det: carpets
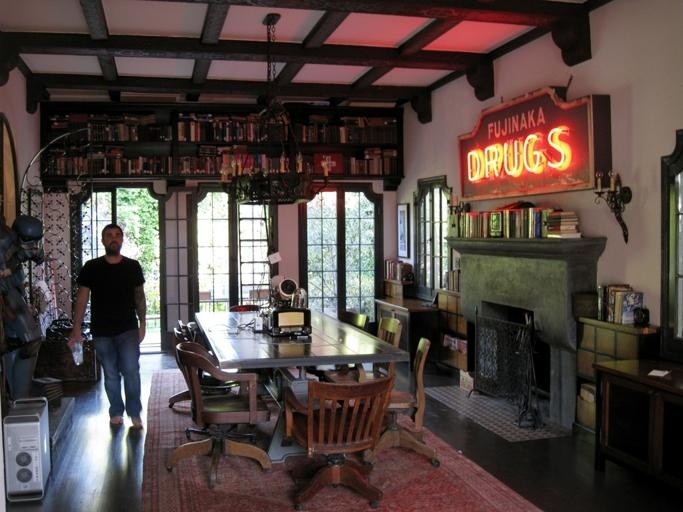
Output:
[140,368,544,512]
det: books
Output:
[443,270,459,293]
[48,111,399,180]
[384,258,412,282]
[595,284,645,326]
[448,207,581,239]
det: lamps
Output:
[228,14,328,205]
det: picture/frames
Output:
[396,203,410,259]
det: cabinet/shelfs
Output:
[593,360,683,488]
[573,315,658,446]
[375,298,439,372]
[436,287,476,376]
[382,280,416,298]
[41,101,404,180]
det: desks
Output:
[47,328,101,391]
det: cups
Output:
[70,341,83,366]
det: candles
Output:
[610,175,616,191]
[450,195,458,206]
[597,177,602,191]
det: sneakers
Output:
[131,416,143,430]
[110,416,123,425]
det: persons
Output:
[66,223,147,433]
